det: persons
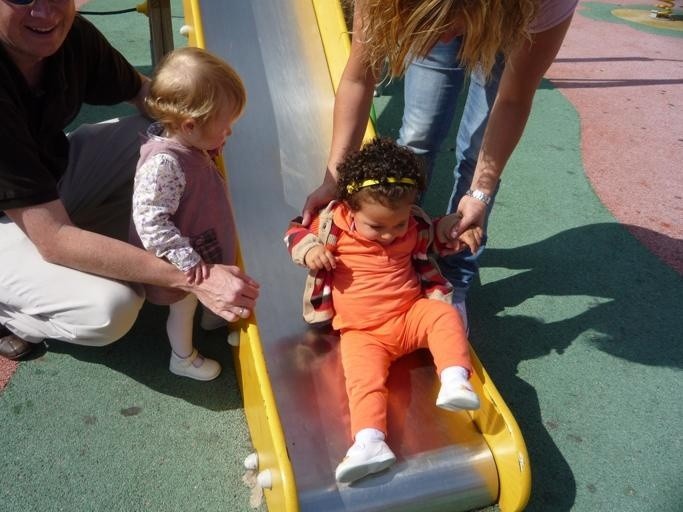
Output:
[279,135,487,486]
[114,45,248,384]
[298,1,580,341]
[0,0,262,378]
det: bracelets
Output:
[465,187,491,206]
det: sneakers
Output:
[452,298,471,340]
[333,437,397,486]
[435,380,483,413]
[0,333,36,359]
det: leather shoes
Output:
[166,347,223,382]
[198,316,227,333]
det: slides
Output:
[179,0,532,510]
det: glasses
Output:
[3,0,35,6]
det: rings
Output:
[237,307,244,317]
